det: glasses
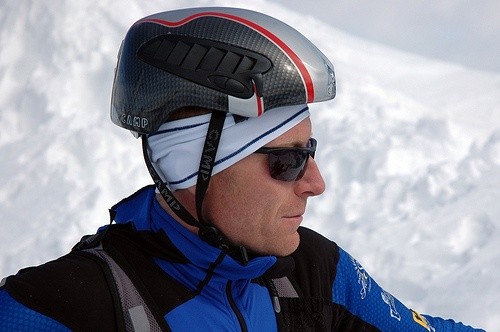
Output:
[247,139,318,183]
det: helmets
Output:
[110,6,338,132]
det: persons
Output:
[0,5,484,332]
